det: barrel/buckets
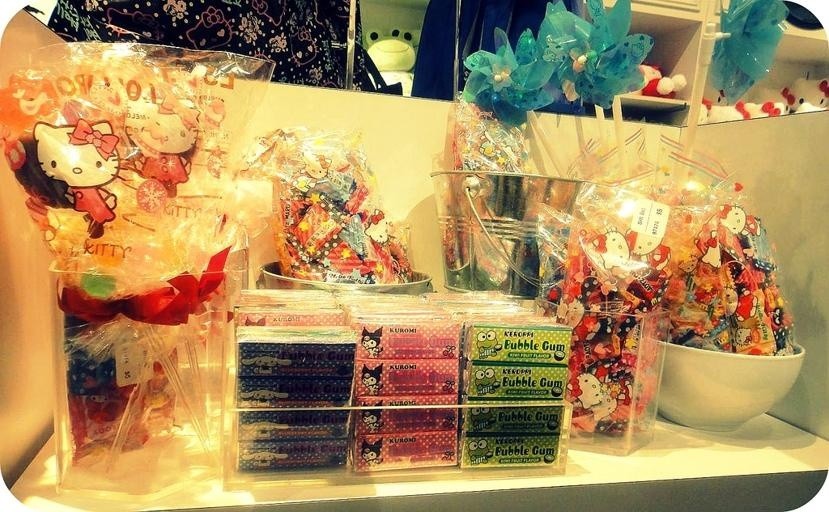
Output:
[430,170,582,303]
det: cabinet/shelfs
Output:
[579,0,828,125]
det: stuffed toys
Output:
[780,76,829,114]
[698,98,791,125]
[639,63,688,99]
[364,26,422,97]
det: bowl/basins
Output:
[655,339,805,431]
